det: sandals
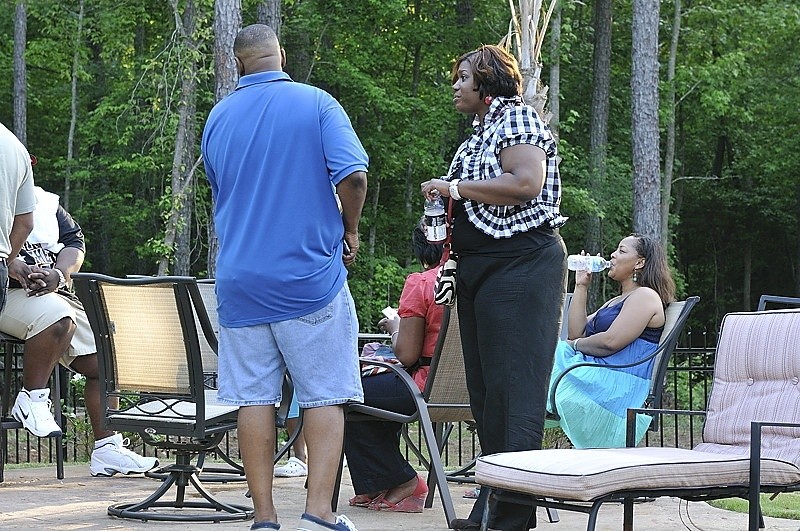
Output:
[273,457,307,477]
[463,490,480,499]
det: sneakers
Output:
[296,513,359,531]
[250,523,281,531]
[9,386,62,437]
[90,434,160,476]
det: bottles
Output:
[567,255,611,272]
[424,190,447,244]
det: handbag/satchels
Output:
[433,251,459,306]
[360,342,408,377]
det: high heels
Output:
[367,474,429,513]
[348,490,389,506]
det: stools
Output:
[0,334,65,481]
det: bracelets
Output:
[573,338,581,351]
[391,331,400,342]
[449,179,463,200]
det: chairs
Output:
[71,265,800,531]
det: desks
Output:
[303,335,390,515]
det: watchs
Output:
[53,268,66,288]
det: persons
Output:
[0,153,160,477]
[544,233,676,449]
[201,23,369,531]
[0,122,36,317]
[344,219,444,512]
[421,46,568,531]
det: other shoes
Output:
[451,518,481,531]
